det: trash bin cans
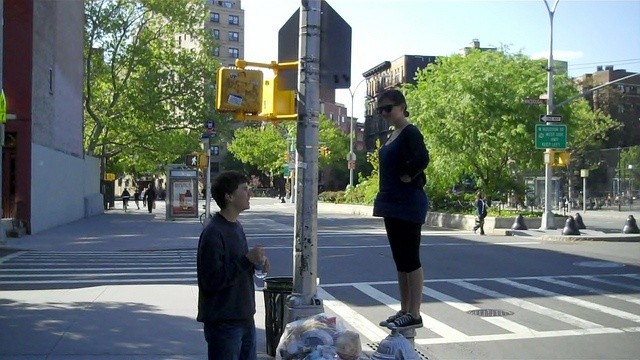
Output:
[263,276,294,357]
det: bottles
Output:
[252,260,267,292]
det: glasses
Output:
[375,104,395,114]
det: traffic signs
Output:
[538,114,563,124]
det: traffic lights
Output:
[184,154,198,167]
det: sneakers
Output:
[472,228,476,234]
[380,310,407,327]
[481,234,486,236]
[387,313,423,331]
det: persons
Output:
[195,171,267,360]
[371,89,430,330]
[121,182,156,216]
[608,191,625,206]
[473,191,487,235]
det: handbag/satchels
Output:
[475,218,482,225]
